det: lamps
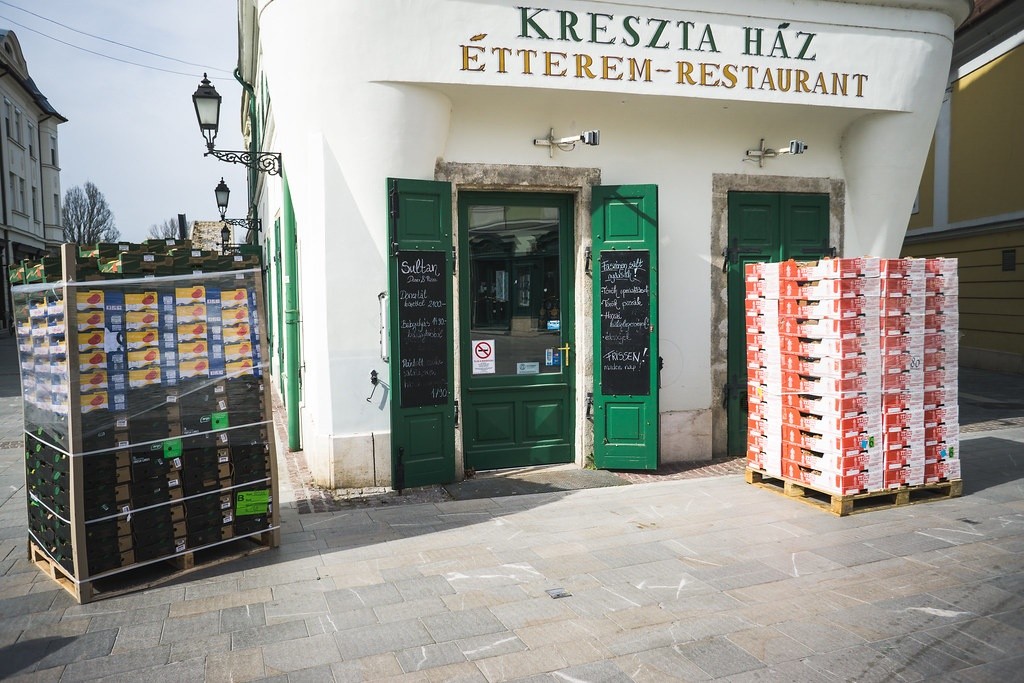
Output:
[535,129,601,149]
[193,72,282,175]
[746,138,809,166]
[220,223,240,255]
[214,177,262,233]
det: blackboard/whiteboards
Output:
[398,251,449,407]
[600,250,650,395]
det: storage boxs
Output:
[742,254,960,495]
[7,237,274,576]
